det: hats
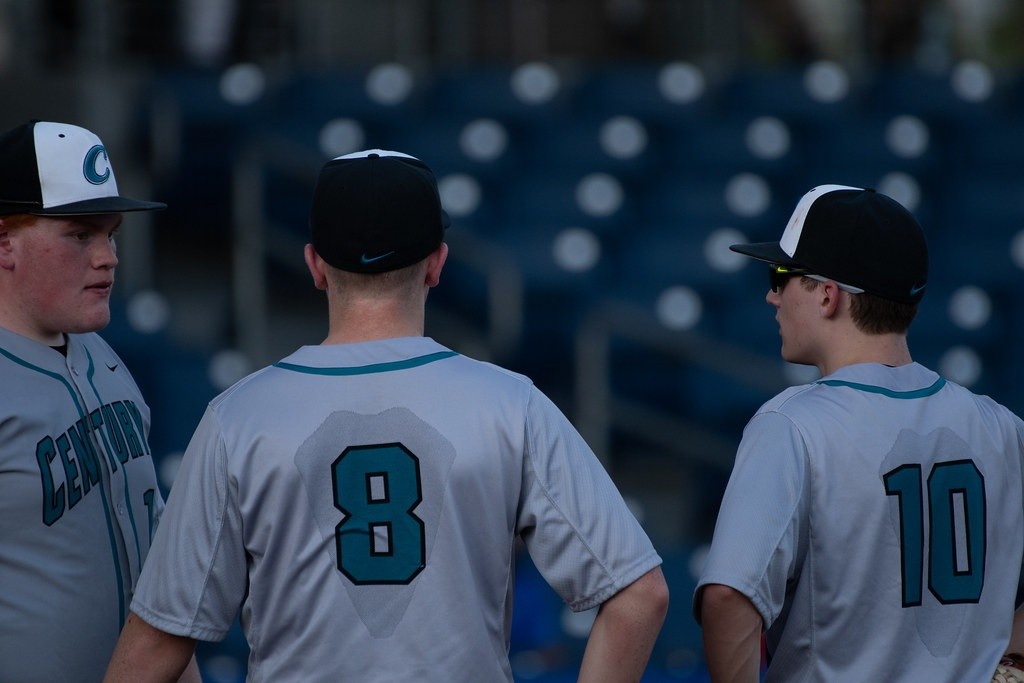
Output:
[730,182,928,305]
[0,119,167,215]
[309,149,448,273]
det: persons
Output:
[690,184,1024,683]
[0,122,202,683]
[102,149,670,683]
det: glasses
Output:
[767,262,817,294]
